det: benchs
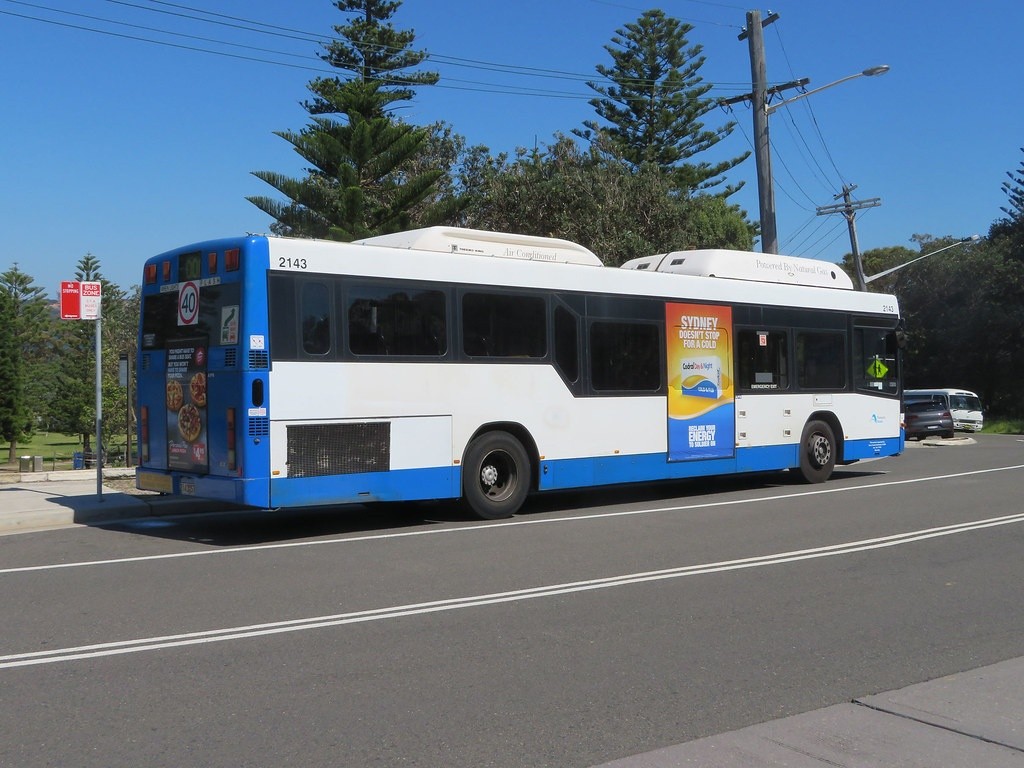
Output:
[73,452,107,469]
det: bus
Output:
[134,226,915,514]
[903,390,984,433]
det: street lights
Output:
[752,65,890,253]
[855,235,982,293]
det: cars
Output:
[902,399,954,441]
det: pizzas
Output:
[167,380,183,410]
[178,404,201,441]
[190,373,206,406]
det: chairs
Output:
[364,333,491,355]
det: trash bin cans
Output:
[73,453,85,470]
[19,456,30,472]
[32,455,43,472]
